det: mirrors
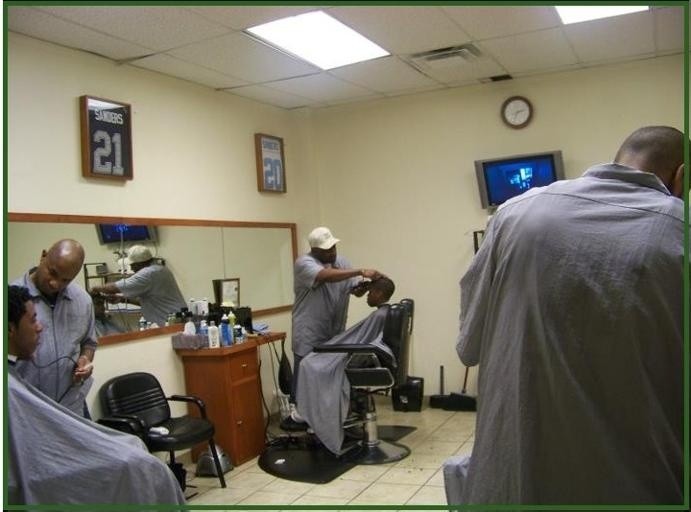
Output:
[8,211,298,348]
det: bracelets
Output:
[361,266,365,274]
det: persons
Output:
[292,227,382,439]
[8,286,43,367]
[444,125,691,512]
[367,277,395,306]
[9,238,100,420]
[92,293,105,320]
[92,244,187,328]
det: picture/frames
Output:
[252,131,289,196]
[78,94,134,180]
[217,278,241,311]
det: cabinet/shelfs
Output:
[80,256,167,315]
[173,330,289,468]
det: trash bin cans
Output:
[391,377,424,411]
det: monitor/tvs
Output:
[474,150,562,208]
[95,223,156,245]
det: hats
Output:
[307,226,339,251]
[117,245,153,264]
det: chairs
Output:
[99,371,229,491]
[282,296,416,464]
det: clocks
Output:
[500,94,535,131]
